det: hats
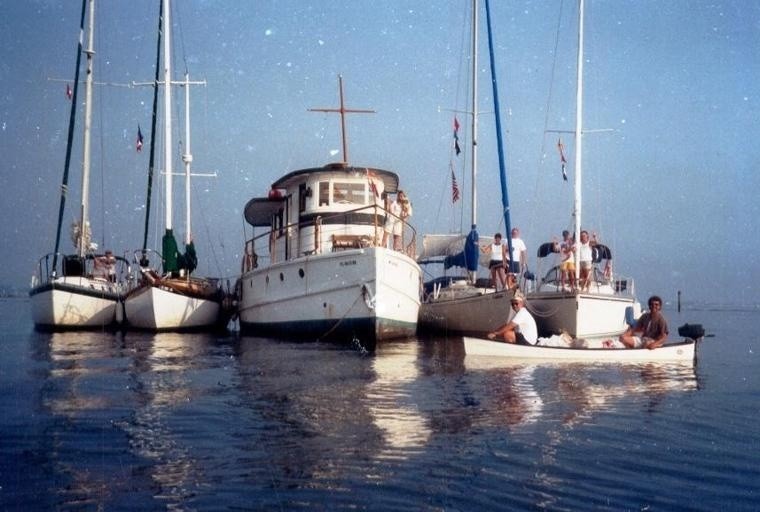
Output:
[511,294,524,301]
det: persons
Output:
[619,296,669,349]
[553,230,576,292]
[95,249,116,283]
[480,233,508,293]
[488,296,538,345]
[382,191,409,251]
[504,228,526,288]
[478,361,677,437]
[572,231,598,292]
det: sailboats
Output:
[416,0,533,339]
[23,0,123,332]
[121,0,229,333]
[525,1,645,341]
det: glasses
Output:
[511,302,517,306]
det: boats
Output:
[230,73,422,355]
[460,334,696,364]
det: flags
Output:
[136,124,143,151]
[454,118,460,129]
[562,165,569,181]
[67,83,74,99]
[558,141,568,163]
[450,165,459,204]
[370,178,380,199]
[453,130,460,139]
[453,141,461,155]
